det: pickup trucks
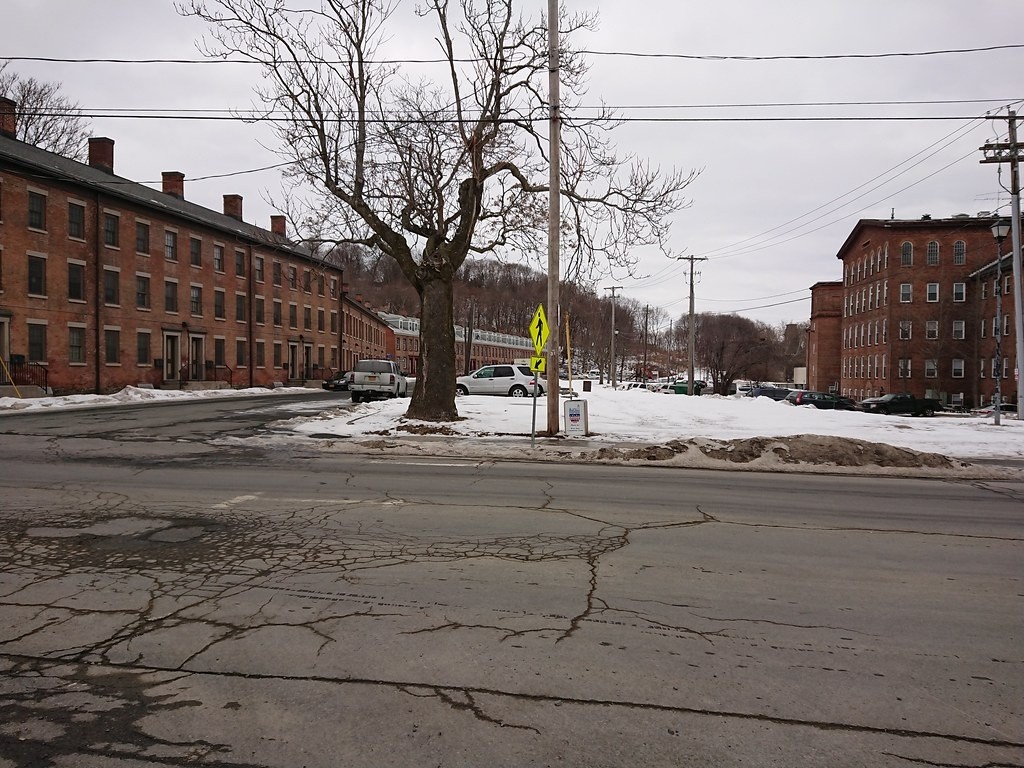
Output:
[857,393,944,417]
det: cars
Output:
[970,403,1017,417]
[455,364,546,398]
[628,383,675,394]
[747,388,857,411]
[322,358,408,402]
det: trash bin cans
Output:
[583,381,591,392]
[674,382,688,394]
[564,400,588,436]
